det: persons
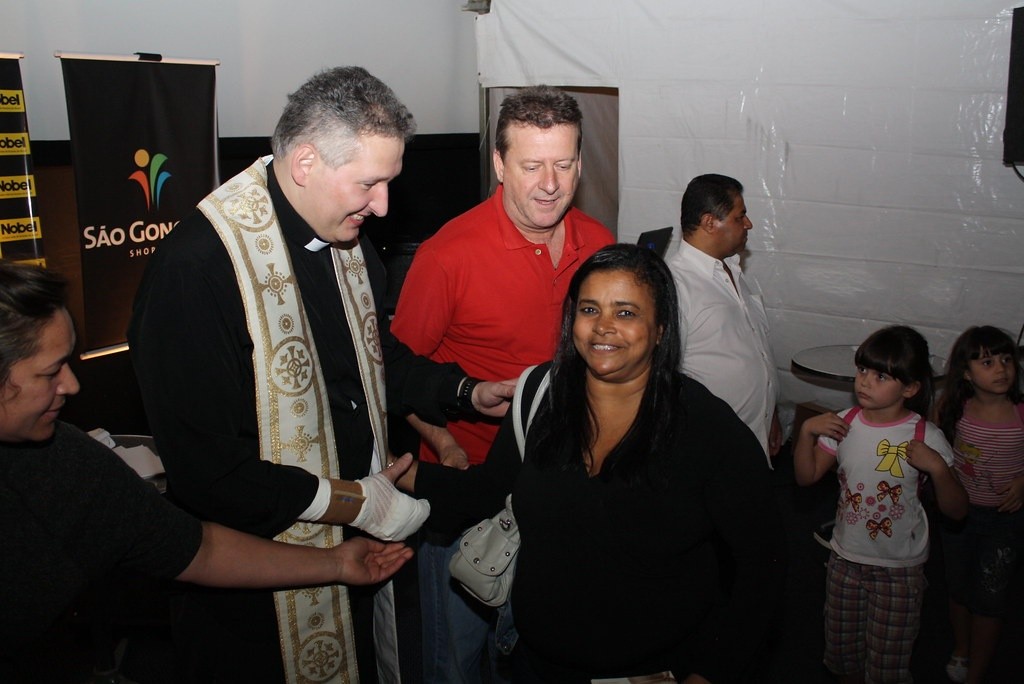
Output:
[932,326,1024,684]
[391,90,616,684]
[0,259,414,684]
[384,245,773,684]
[794,324,963,684]
[661,173,786,466]
[127,70,519,684]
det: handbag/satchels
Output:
[449,497,520,607]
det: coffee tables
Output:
[791,345,952,384]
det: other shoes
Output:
[947,655,970,682]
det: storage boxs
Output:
[790,398,845,446]
